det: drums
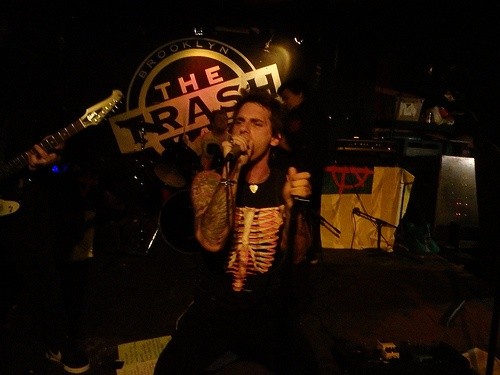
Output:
[159,190,204,255]
[152,139,200,188]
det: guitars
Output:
[0,90,125,180]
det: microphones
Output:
[226,145,242,162]
